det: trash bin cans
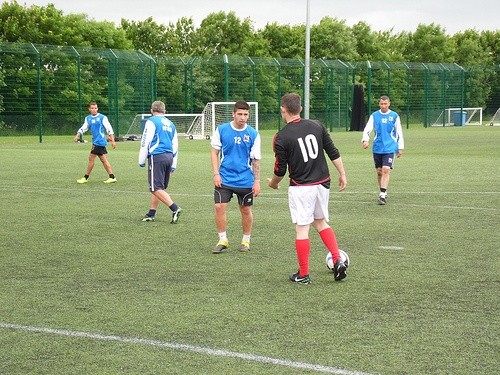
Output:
[454,111,467,126]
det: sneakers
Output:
[334,258,346,281]
[170,207,181,224]
[77,177,88,183]
[378,197,386,204]
[289,271,311,285]
[141,214,154,221]
[240,241,249,253]
[212,241,228,253]
[104,178,117,184]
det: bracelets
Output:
[213,174,219,176]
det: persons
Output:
[361,95,404,205]
[211,100,261,253]
[138,101,182,224]
[74,101,117,184]
[267,93,347,285]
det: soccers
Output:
[326,249,350,273]
